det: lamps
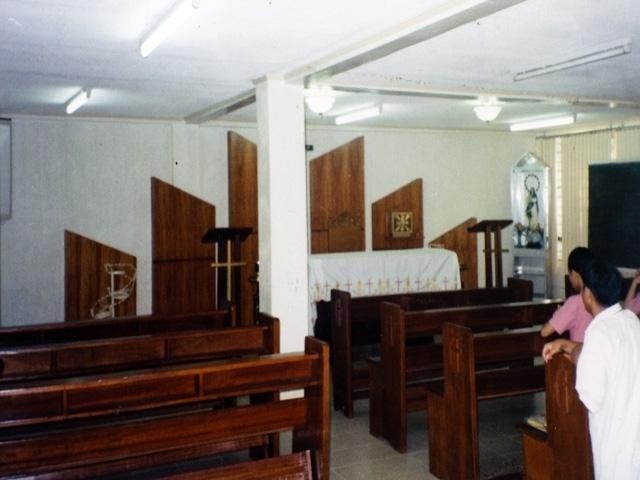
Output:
[509,111,575,132]
[139,0,196,58]
[334,101,382,125]
[65,84,92,115]
[473,106,502,124]
[513,37,631,82]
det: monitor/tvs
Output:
[586,160,640,280]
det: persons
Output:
[525,187,539,232]
[540,247,640,480]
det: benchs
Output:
[0,274,640,480]
